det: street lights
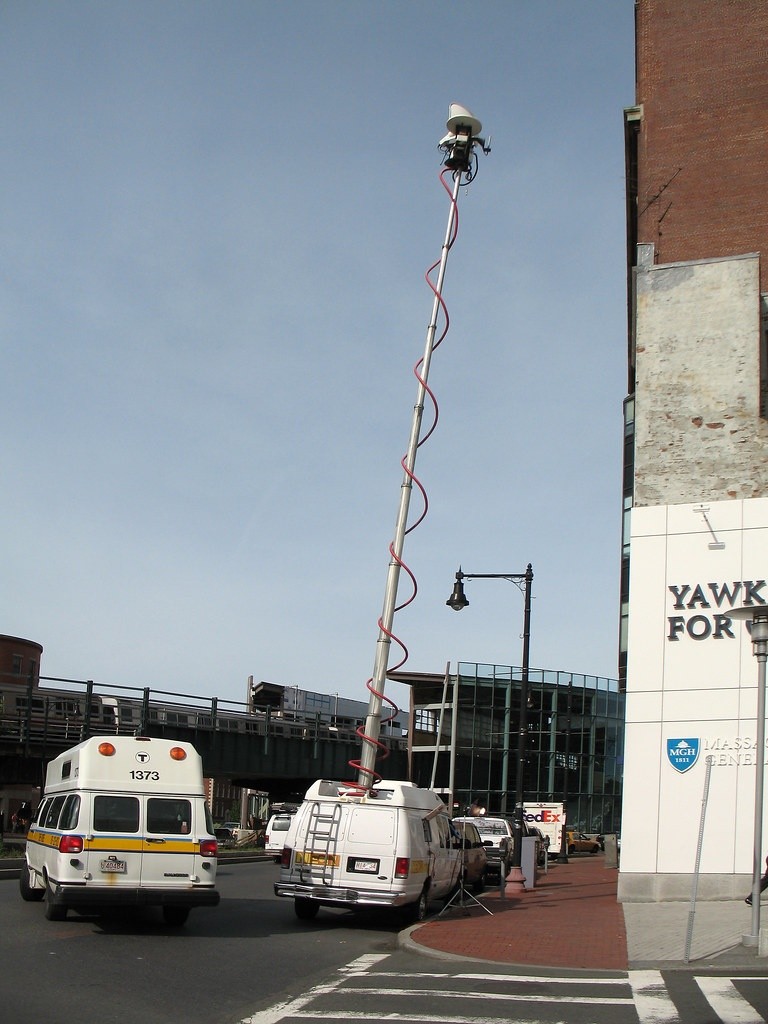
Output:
[447,571,535,823]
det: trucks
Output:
[515,801,567,859]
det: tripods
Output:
[435,807,494,917]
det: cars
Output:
[214,828,236,849]
[601,834,621,851]
[222,822,240,838]
[565,832,602,854]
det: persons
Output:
[11,812,18,833]
[745,857,768,907]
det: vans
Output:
[262,813,295,863]
[20,733,221,923]
[273,779,471,926]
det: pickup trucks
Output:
[452,816,516,873]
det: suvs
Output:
[449,822,494,893]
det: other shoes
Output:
[745,898,752,905]
